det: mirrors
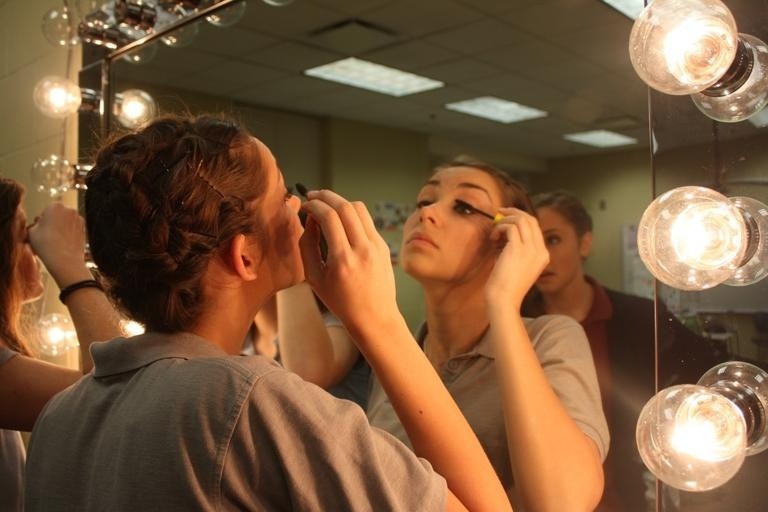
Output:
[75,0,768,511]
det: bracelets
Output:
[56,280,105,303]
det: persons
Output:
[365,151,612,512]
[522,188,715,511]
[21,109,513,511]
[237,277,372,414]
[0,178,130,512]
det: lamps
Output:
[262,1,296,7]
[689,33,768,124]
[635,186,750,292]
[635,384,755,492]
[720,196,768,286]
[205,1,248,28]
[628,0,743,97]
[695,361,768,457]
